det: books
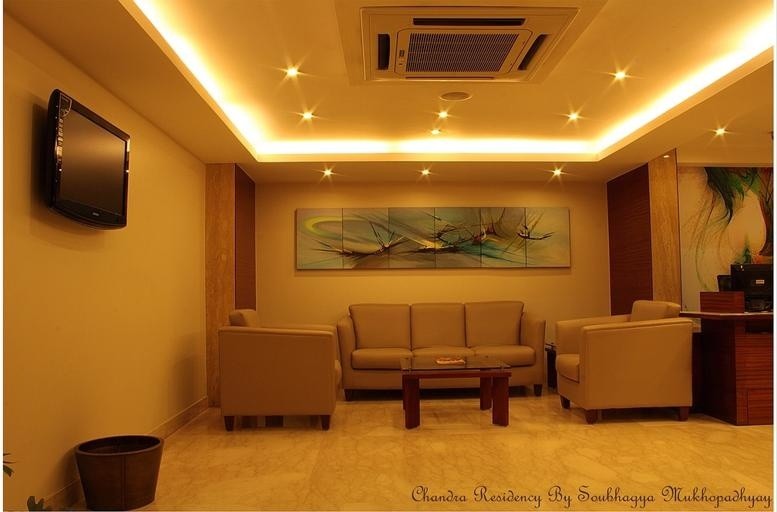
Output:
[434,359,465,364]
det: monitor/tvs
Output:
[46,89,131,231]
[731,263,773,312]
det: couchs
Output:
[337,300,546,401]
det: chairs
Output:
[554,299,693,425]
[217,308,341,429]
